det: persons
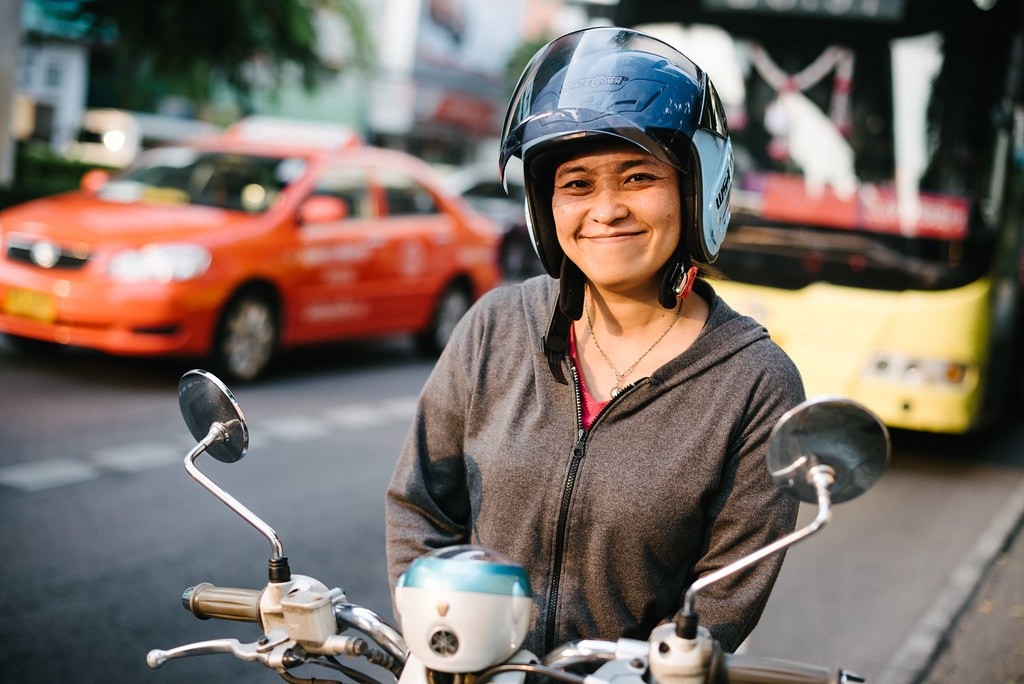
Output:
[379,25,808,684]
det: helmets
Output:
[521,52,735,276]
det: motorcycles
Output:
[144,369,889,684]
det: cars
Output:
[0,118,508,383]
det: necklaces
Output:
[583,278,683,403]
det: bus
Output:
[608,1,1023,435]
